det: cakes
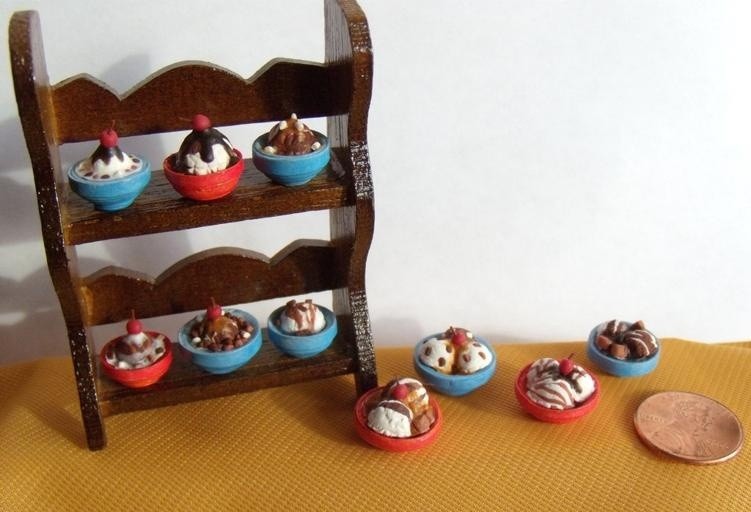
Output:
[106,310,166,369]
[171,115,237,174]
[365,375,434,438]
[525,357,596,408]
[274,299,326,336]
[264,113,320,156]
[596,318,658,359]
[191,297,252,350]
[75,119,142,181]
[420,327,492,374]
